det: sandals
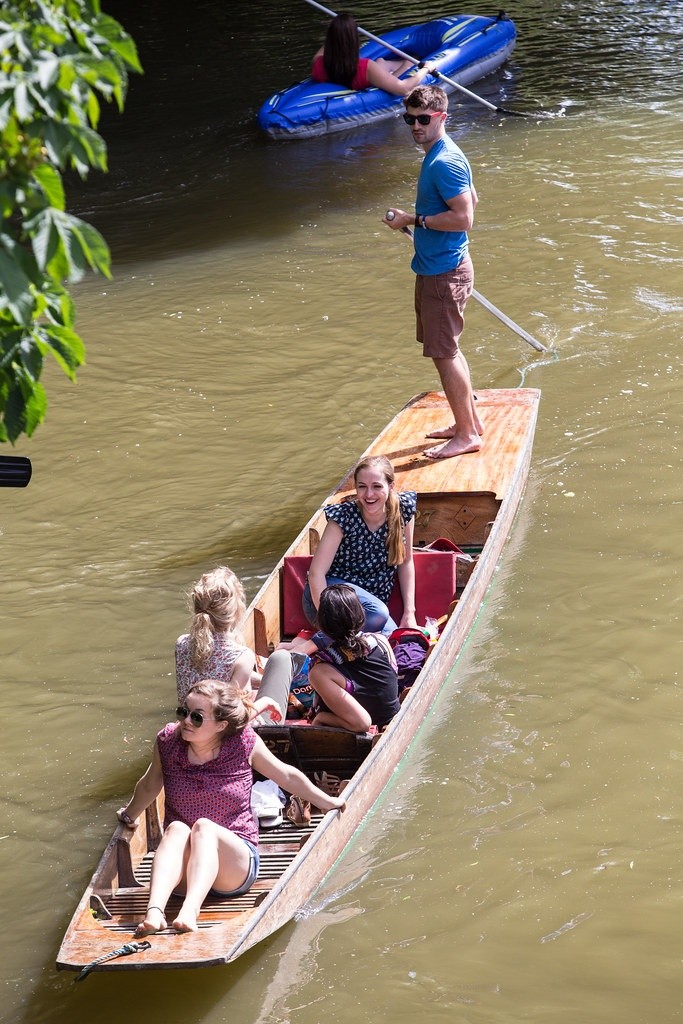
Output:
[285,795,311,828]
[314,771,341,814]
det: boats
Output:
[54,386,542,969]
[256,14,518,139]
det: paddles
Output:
[304,0,535,118]
[386,210,547,352]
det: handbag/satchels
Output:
[389,627,429,687]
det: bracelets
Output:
[422,215,428,230]
[414,213,423,228]
[423,67,430,73]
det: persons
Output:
[309,13,437,95]
[175,566,295,727]
[275,455,417,655]
[309,584,402,734]
[115,679,347,933]
[380,85,485,459]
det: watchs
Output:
[121,810,134,824]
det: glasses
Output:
[403,112,442,125]
[176,706,220,728]
